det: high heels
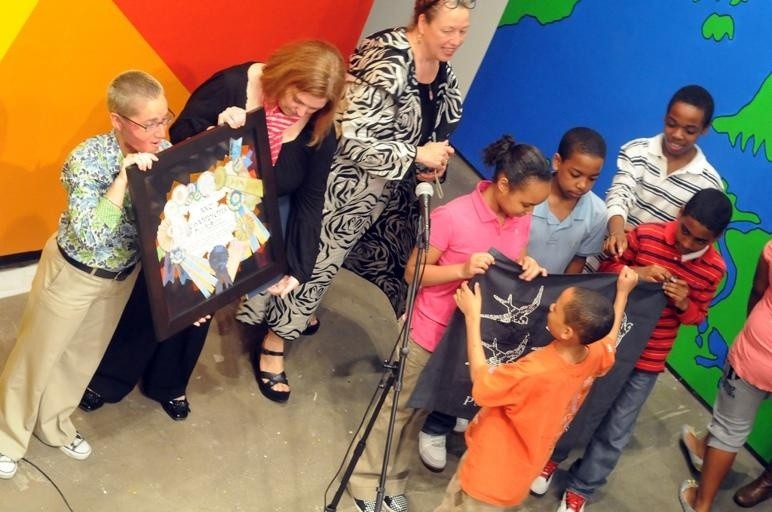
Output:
[252,338,291,402]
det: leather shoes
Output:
[79,388,104,412]
[162,394,190,420]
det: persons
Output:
[530,188,733,512]
[79,40,346,420]
[734,460,772,508]
[680,240,772,511]
[343,132,550,512]
[0,68,175,478]
[431,266,638,512]
[237,0,476,403]
[420,126,606,472]
[603,84,726,258]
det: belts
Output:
[57,241,142,282]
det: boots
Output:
[733,458,770,508]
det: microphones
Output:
[415,182,433,250]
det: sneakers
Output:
[418,429,447,470]
[0,453,19,480]
[555,489,587,512]
[453,417,470,434]
[58,430,92,460]
[353,495,387,512]
[679,478,700,512]
[383,494,411,512]
[531,459,557,496]
[681,422,703,472]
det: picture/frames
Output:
[126,105,290,343]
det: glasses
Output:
[116,107,176,132]
[420,0,477,13]
